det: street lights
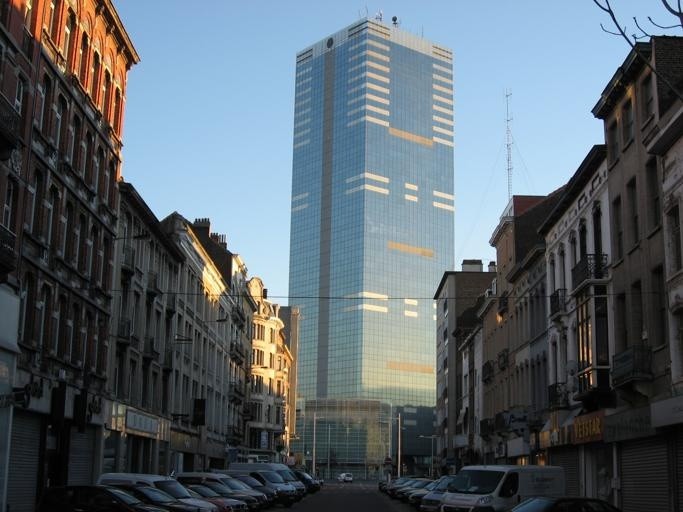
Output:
[416,433,437,479]
[377,421,390,463]
[312,411,325,478]
[387,412,401,479]
[326,425,336,482]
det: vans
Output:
[336,473,354,483]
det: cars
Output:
[378,463,621,512]
[40,459,325,512]
